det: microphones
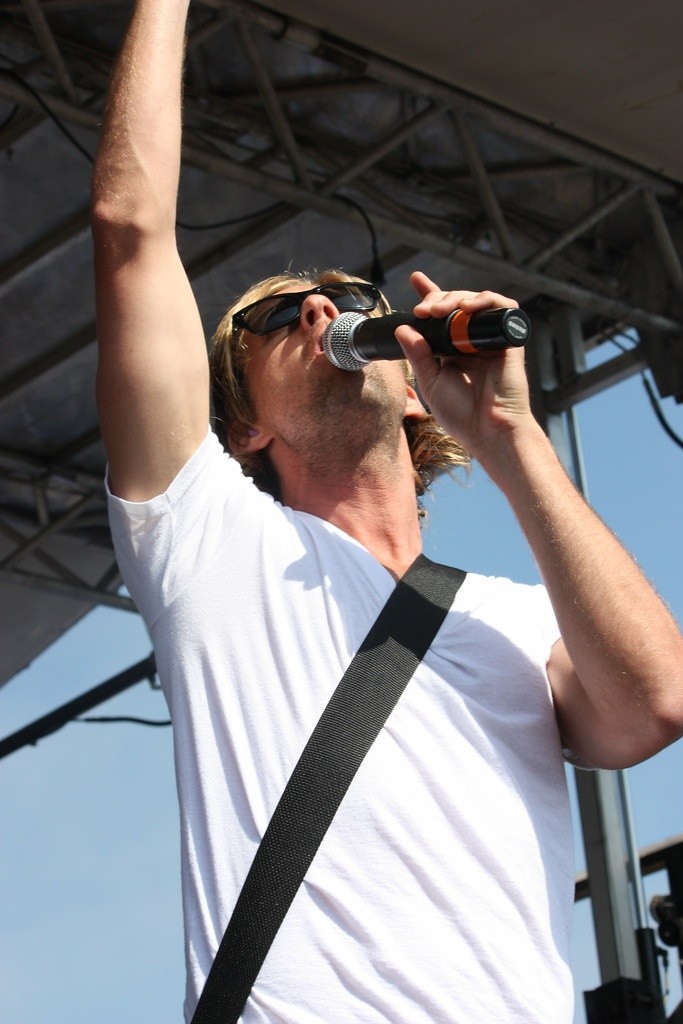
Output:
[322,311,529,371]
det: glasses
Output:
[230,281,387,374]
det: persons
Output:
[91,1,683,1023]
